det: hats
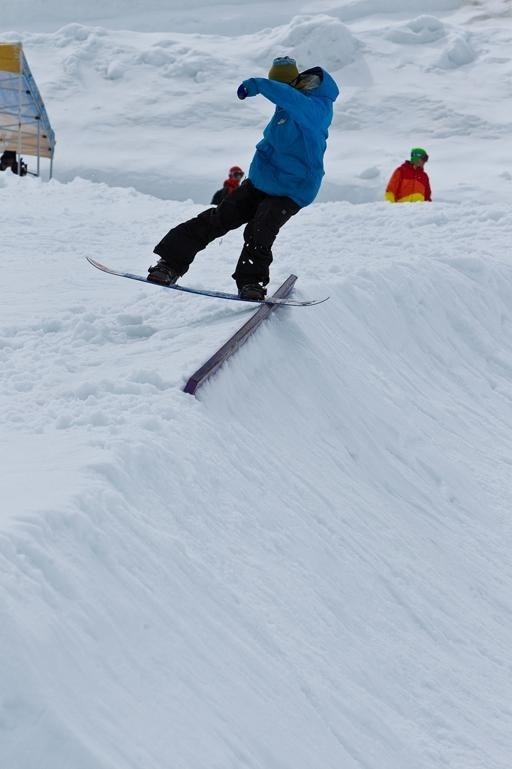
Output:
[228,167,243,179]
[268,56,299,85]
[411,148,427,164]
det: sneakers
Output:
[147,262,175,285]
[238,281,267,301]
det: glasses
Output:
[232,172,244,178]
[419,154,428,162]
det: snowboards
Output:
[86,256,329,306]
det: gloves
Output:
[237,84,247,101]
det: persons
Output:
[147,54,340,301]
[210,166,244,205]
[384,147,432,203]
